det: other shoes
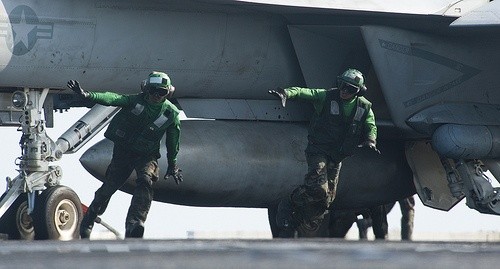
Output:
[126,225,144,237]
[81,215,94,238]
[276,198,295,228]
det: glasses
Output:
[340,82,356,93]
[150,86,167,95]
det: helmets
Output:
[336,69,367,97]
[141,71,172,97]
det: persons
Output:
[264,69,381,234]
[368,196,415,242]
[66,70,184,239]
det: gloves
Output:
[67,79,87,99]
[357,139,382,160]
[164,165,184,184]
[265,87,287,108]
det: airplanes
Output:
[0,0,500,243]
